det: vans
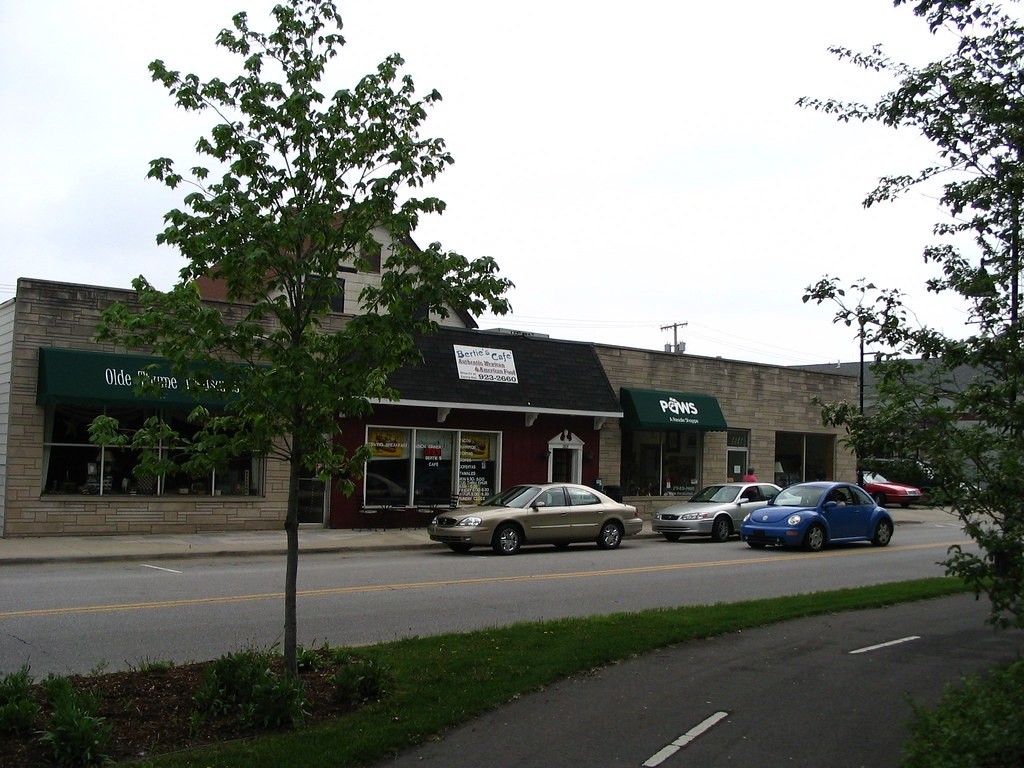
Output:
[858,459,939,504]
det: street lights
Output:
[858,314,868,412]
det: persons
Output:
[827,488,847,508]
[741,466,759,494]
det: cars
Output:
[298,473,408,521]
[858,470,922,507]
[651,482,802,542]
[428,482,643,556]
[740,480,894,553]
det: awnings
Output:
[33,348,280,420]
[619,387,727,435]
